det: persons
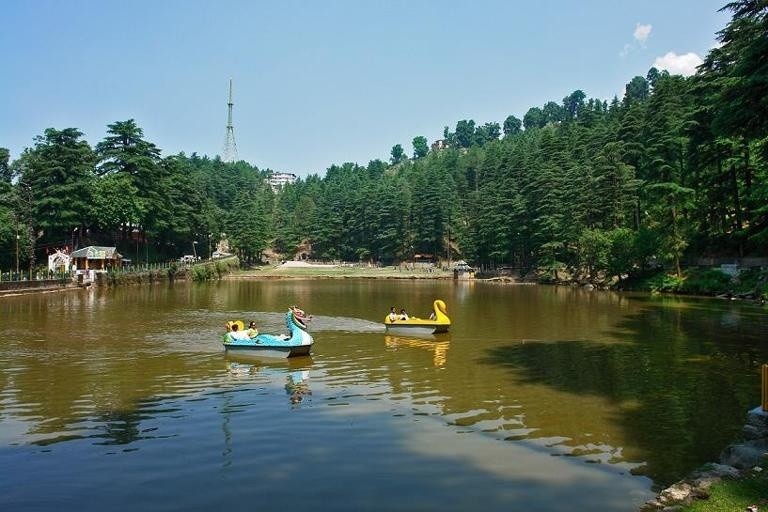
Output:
[247,321,258,339]
[389,307,397,321]
[429,308,437,321]
[399,309,409,320]
[229,324,250,340]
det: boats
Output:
[383,299,452,334]
[221,305,314,358]
[225,354,313,411]
[385,334,451,370]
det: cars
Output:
[212,252,221,259]
